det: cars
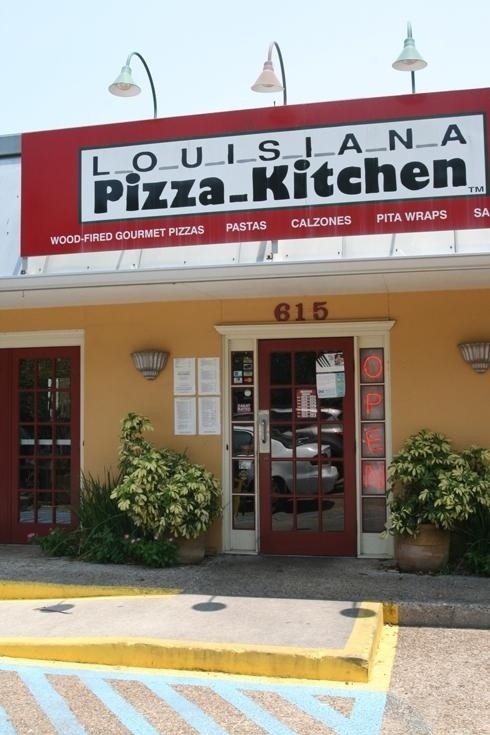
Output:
[232,405,344,485]
[233,425,339,514]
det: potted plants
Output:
[377,427,489,572]
[108,411,224,566]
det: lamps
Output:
[108,50,159,119]
[250,39,287,105]
[392,21,428,93]
